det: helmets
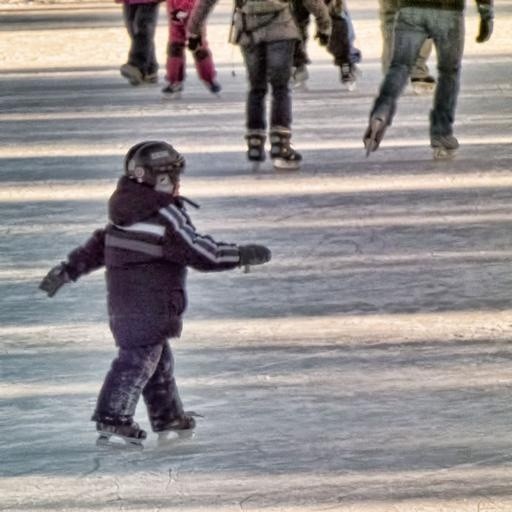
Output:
[124,141,185,195]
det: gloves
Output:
[39,261,71,297]
[239,244,271,273]
[476,3,495,43]
[313,29,332,46]
[188,33,203,50]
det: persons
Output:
[38,139,272,452]
[377,0,437,96]
[288,1,362,92]
[162,0,223,97]
[116,0,162,86]
[227,1,310,171]
[362,0,495,161]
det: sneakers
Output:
[288,63,309,84]
[363,102,392,152]
[432,133,459,150]
[270,132,302,162]
[152,414,196,433]
[143,72,158,83]
[96,421,148,439]
[411,77,436,87]
[247,135,266,160]
[162,82,185,93]
[339,60,359,82]
[207,81,222,92]
[120,64,142,86]
